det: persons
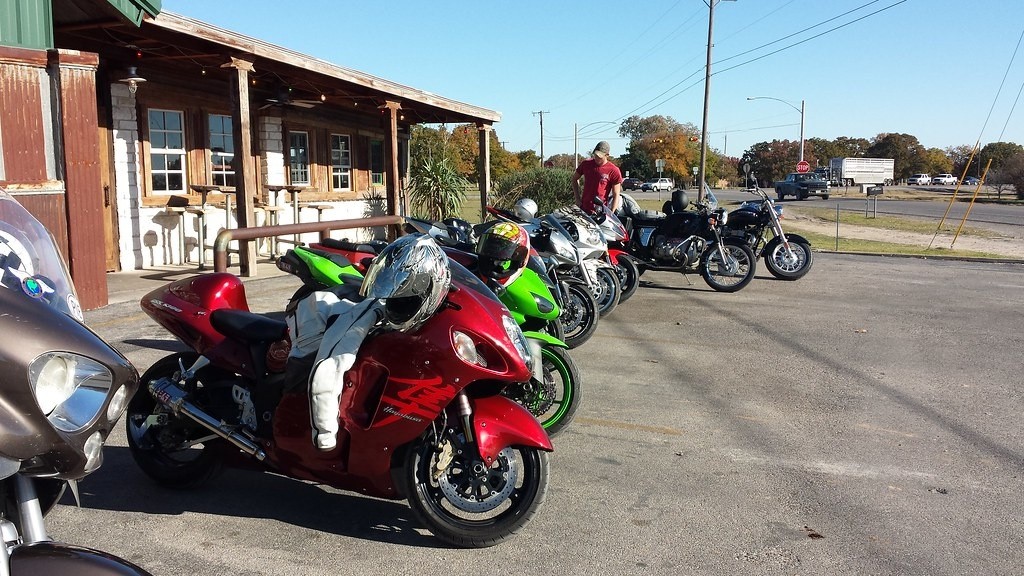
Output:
[571,140,622,216]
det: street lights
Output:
[574,120,617,180]
[748,95,812,169]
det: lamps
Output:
[118,66,148,94]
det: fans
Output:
[257,93,324,110]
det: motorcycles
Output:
[278,161,815,447]
[124,234,556,549]
[0,191,155,574]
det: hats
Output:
[592,141,610,159]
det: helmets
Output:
[672,190,689,210]
[514,198,539,223]
[476,221,530,288]
[358,232,451,335]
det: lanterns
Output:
[689,136,698,142]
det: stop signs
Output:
[795,161,810,173]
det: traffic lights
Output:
[651,138,663,143]
[689,136,697,141]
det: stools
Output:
[166,202,334,264]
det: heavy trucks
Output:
[818,157,895,187]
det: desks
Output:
[190,184,236,267]
[264,185,306,261]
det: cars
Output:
[621,178,644,190]
[961,176,984,186]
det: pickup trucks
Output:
[908,174,936,185]
[932,174,958,185]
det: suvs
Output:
[773,172,830,201]
[642,177,675,192]
